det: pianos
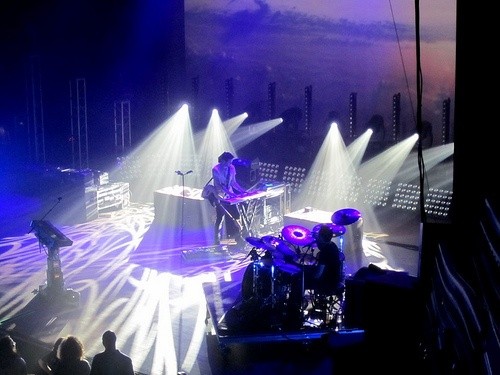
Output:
[219,190,267,251]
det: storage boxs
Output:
[42,170,129,223]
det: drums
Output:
[279,245,297,255]
[242,263,273,300]
[265,250,284,259]
[274,264,302,311]
[292,253,318,266]
[260,259,285,266]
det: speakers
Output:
[231,160,256,193]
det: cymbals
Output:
[331,209,360,225]
[313,223,346,238]
[282,225,314,246]
[262,235,282,249]
[245,237,270,249]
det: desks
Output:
[154,181,334,240]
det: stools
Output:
[314,283,344,325]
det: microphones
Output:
[37,197,62,222]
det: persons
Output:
[287,227,345,323]
[212,152,247,245]
[0,335,27,375]
[38,336,91,375]
[90,330,134,375]
